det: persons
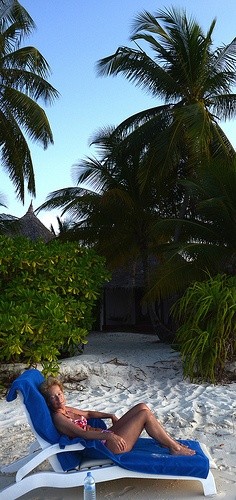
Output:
[40,378,197,456]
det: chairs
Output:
[0,370,219,500]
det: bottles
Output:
[83,472,97,500]
